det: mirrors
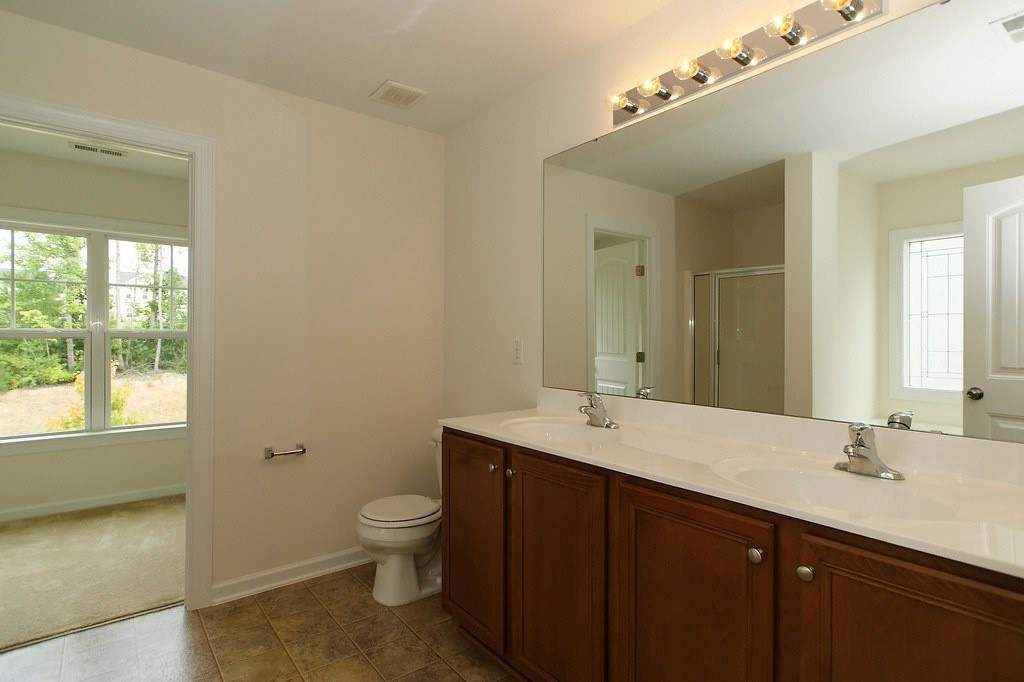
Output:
[541,0,1024,446]
[683,269,713,406]
[709,265,784,415]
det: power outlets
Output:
[512,338,523,365]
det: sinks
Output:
[501,415,648,460]
[711,454,918,518]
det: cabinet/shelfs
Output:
[441,430,607,681]
[607,480,1024,682]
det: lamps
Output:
[762,7,804,48]
[714,30,755,69]
[604,87,640,114]
[636,73,673,101]
[821,0,864,21]
[672,56,711,85]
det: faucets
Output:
[636,386,655,399]
[842,422,904,481]
[886,410,917,431]
[576,392,620,431]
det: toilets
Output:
[354,422,446,610]
